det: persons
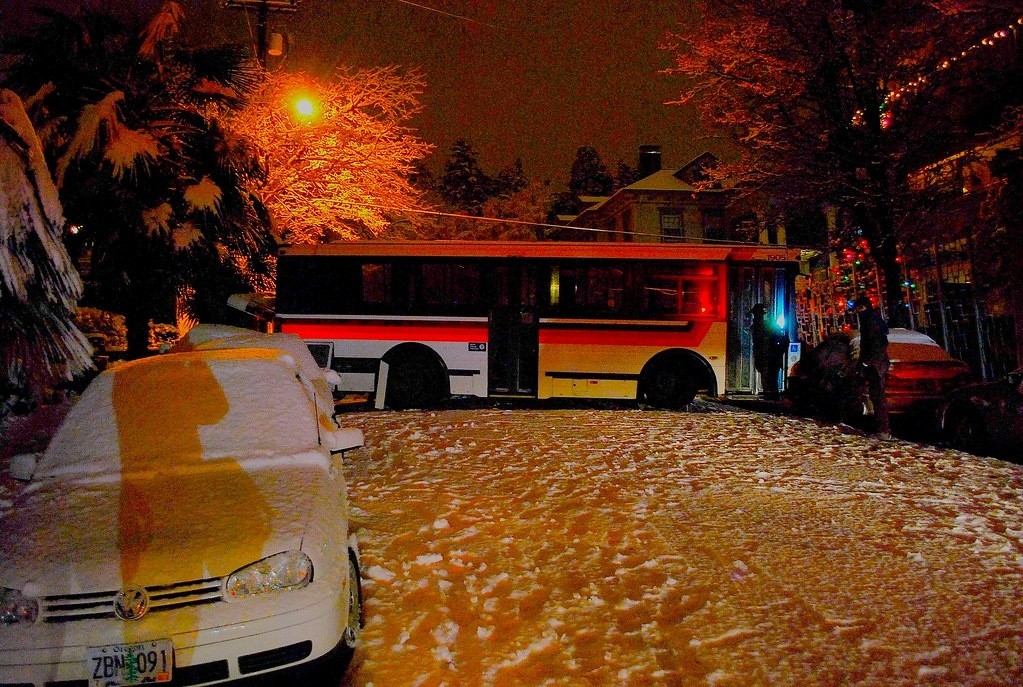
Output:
[750,301,788,402]
[850,297,894,443]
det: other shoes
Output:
[875,433,892,441]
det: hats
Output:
[751,303,768,314]
[853,297,868,308]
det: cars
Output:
[0,345,362,687]
[933,364,1023,459]
[802,328,969,427]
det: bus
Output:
[274,239,812,412]
